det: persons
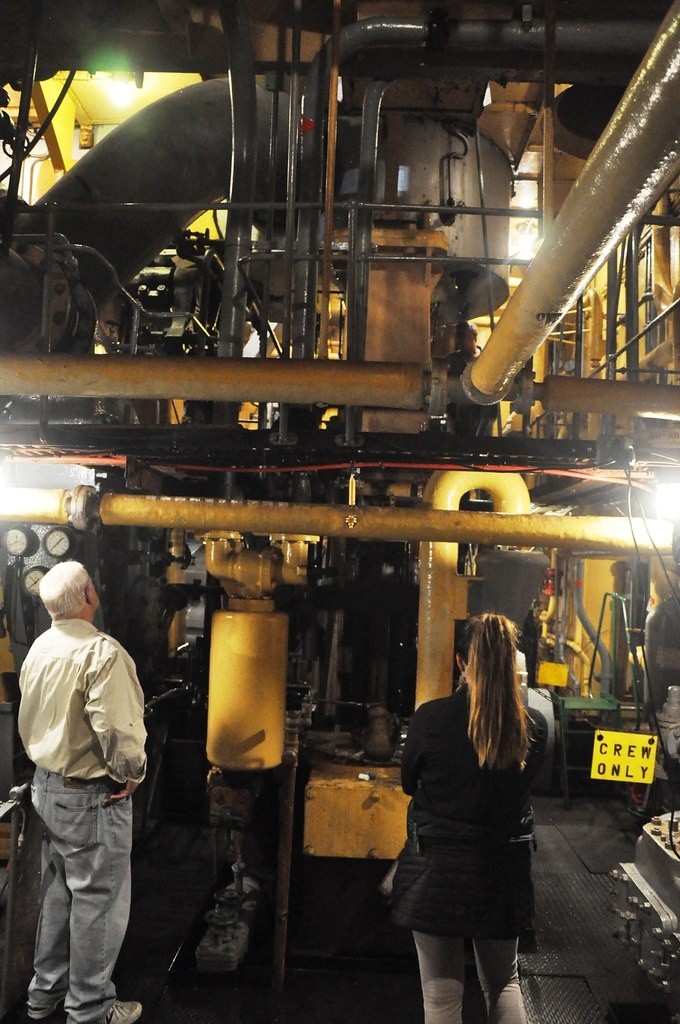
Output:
[17,560,148,1024]
[390,613,549,1024]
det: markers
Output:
[357,769,376,780]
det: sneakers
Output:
[27,995,66,1019]
[66,1000,142,1024]
